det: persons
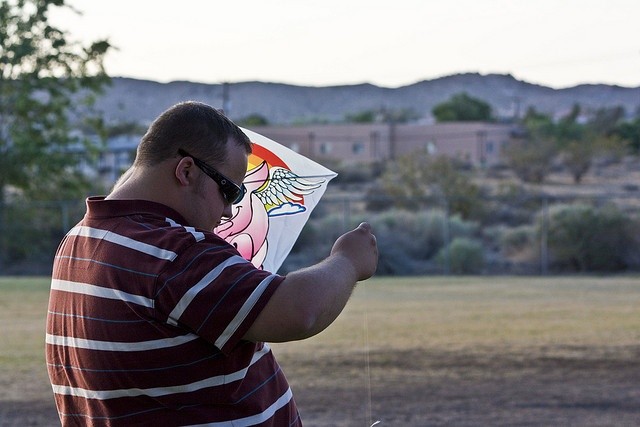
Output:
[46,101,379,426]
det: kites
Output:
[213,124,339,273]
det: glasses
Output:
[176,148,247,203]
